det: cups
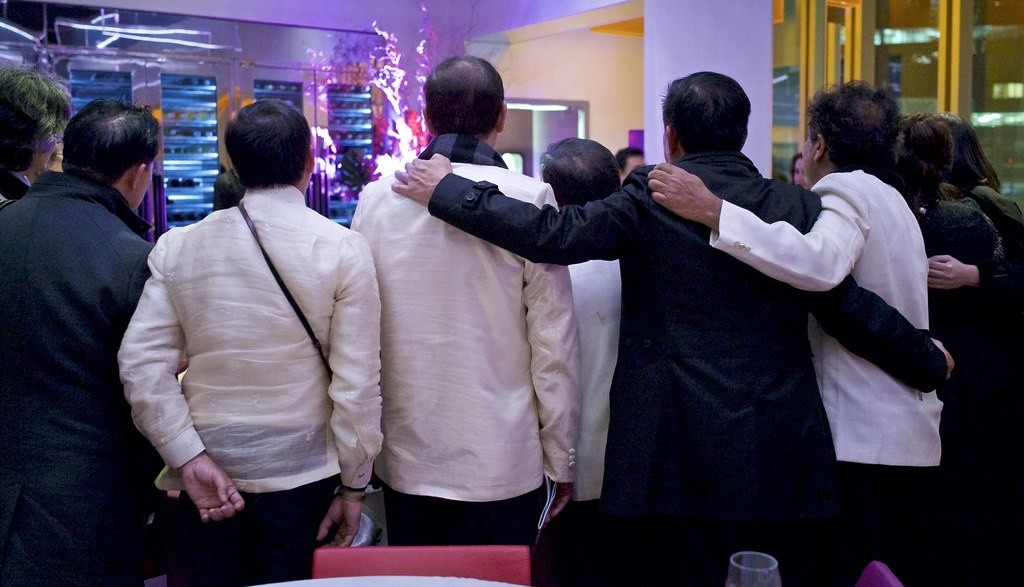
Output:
[726,552,781,587]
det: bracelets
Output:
[337,488,366,501]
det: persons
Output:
[351,56,1024,587]
[117,99,384,587]
[0,68,246,587]
